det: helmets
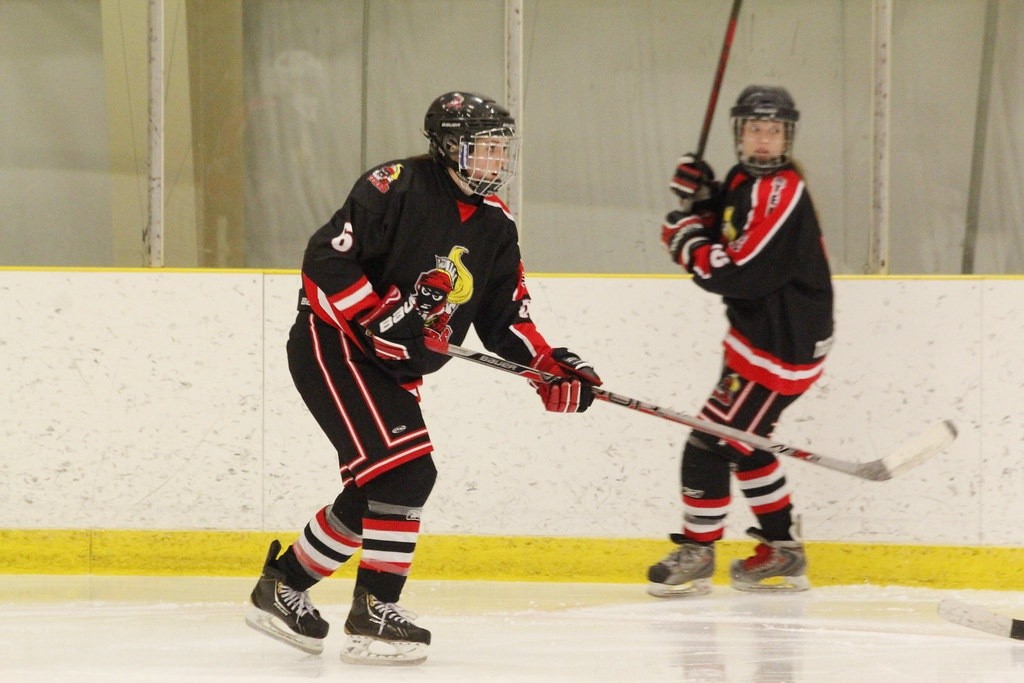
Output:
[424,92,518,195]
[730,85,798,178]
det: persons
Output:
[646,84,835,597]
[245,92,604,666]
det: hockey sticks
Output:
[936,596,1023,642]
[366,325,960,480]
[675,0,745,219]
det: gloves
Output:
[357,283,452,377]
[526,346,603,413]
[667,153,714,205]
[660,211,707,272]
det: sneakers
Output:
[729,524,811,591]
[246,539,329,653]
[645,532,715,596]
[341,585,430,660]
